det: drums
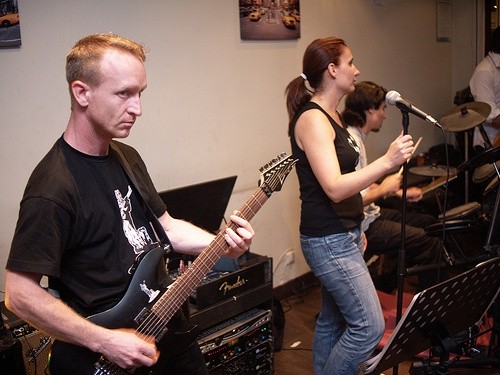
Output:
[465,161,500,201]
[424,200,482,234]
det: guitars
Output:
[47,150,300,375]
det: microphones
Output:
[385,90,442,129]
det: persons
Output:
[285,36,414,375]
[5,32,254,375]
[340,81,444,287]
[469,25,500,146]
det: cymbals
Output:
[437,100,491,132]
[408,164,457,178]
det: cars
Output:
[282,12,300,28]
[250,8,268,21]
[0,12,20,28]
